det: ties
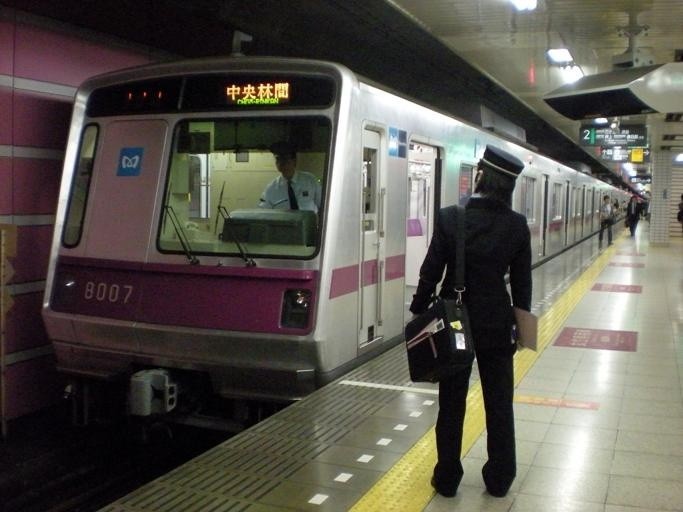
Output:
[288,181,299,210]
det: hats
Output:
[480,144,524,178]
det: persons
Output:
[611,197,651,226]
[627,196,640,236]
[677,194,683,232]
[259,139,322,225]
[409,144,533,498]
[599,195,615,247]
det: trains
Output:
[39,58,642,443]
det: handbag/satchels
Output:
[404,300,475,383]
